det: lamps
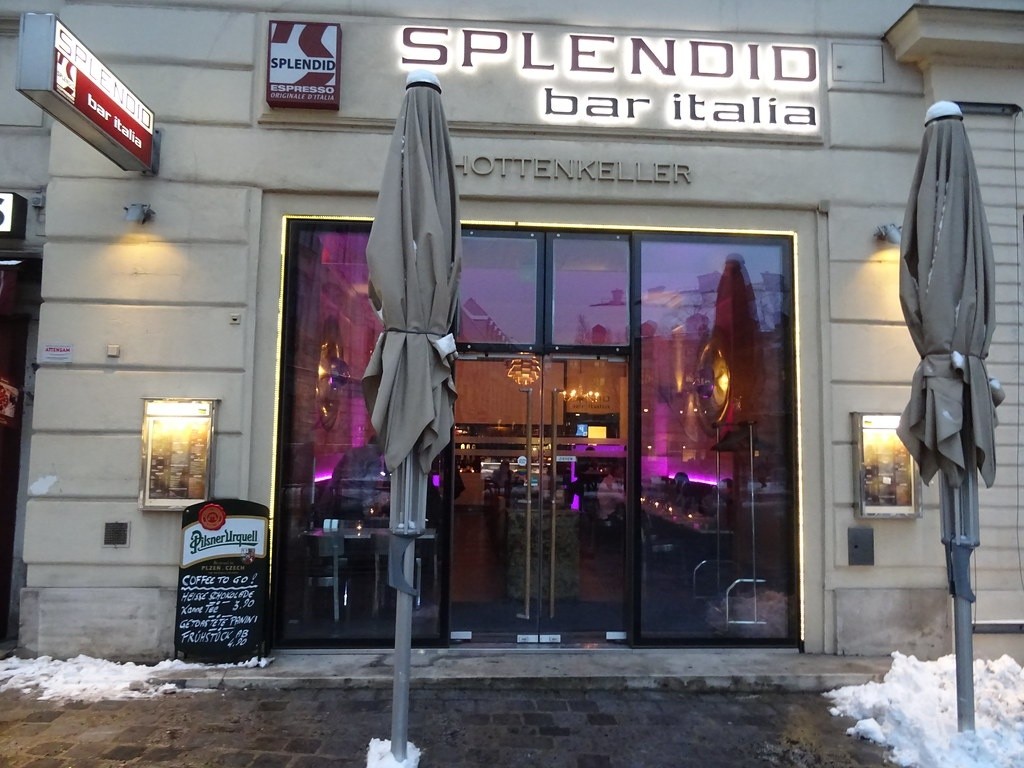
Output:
[505,347,543,386]
[873,221,903,247]
[122,201,155,225]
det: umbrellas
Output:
[902,96,1007,740]
[713,254,770,590]
[359,70,467,766]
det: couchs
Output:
[484,488,583,601]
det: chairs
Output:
[372,531,424,614]
[595,508,623,547]
[616,509,678,587]
[293,529,350,623]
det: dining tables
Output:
[302,527,438,594]
[612,489,736,566]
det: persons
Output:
[331,434,385,520]
[596,463,628,528]
[489,460,527,494]
